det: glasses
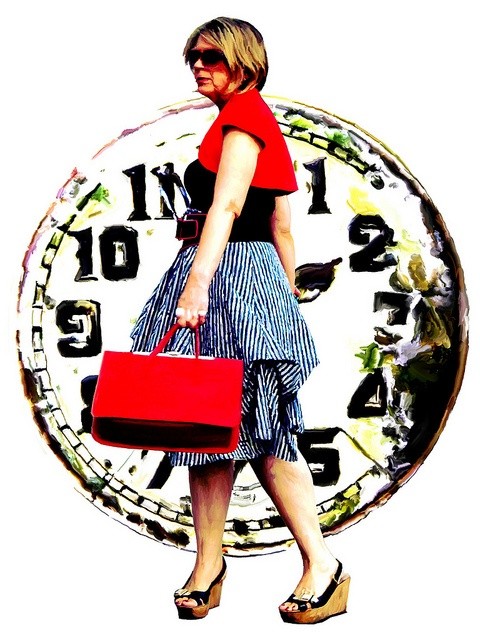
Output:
[188,49,226,68]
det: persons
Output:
[125,15,351,627]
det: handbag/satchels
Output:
[91,323,243,454]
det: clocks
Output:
[15,90,470,556]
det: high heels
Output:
[279,559,351,623]
[174,556,227,620]
